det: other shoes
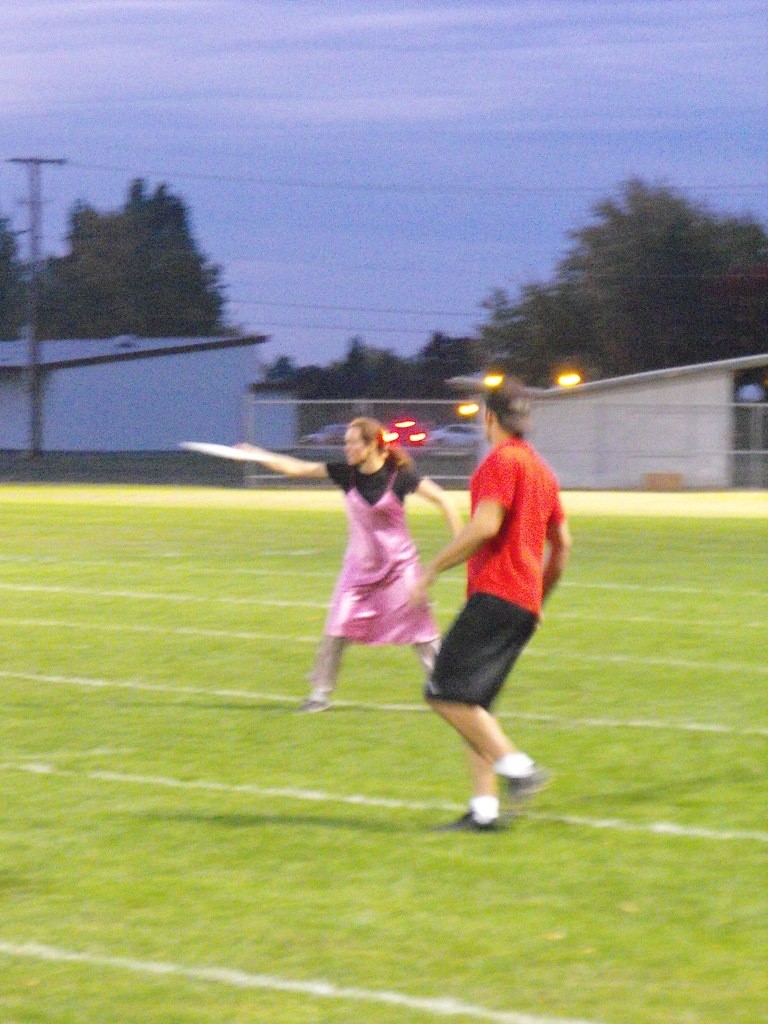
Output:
[432,810,511,835]
[299,701,325,717]
[506,763,554,800]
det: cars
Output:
[426,421,479,449]
[299,421,347,446]
[385,417,429,448]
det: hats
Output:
[486,378,539,434]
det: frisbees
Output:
[179,442,261,464]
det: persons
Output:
[411,373,574,832]
[232,416,464,715]
